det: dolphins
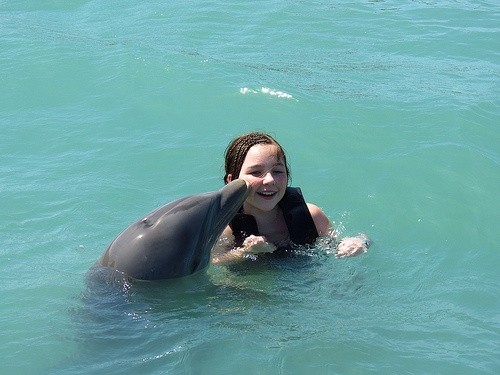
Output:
[73,179,251,375]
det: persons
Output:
[208,134,372,277]
